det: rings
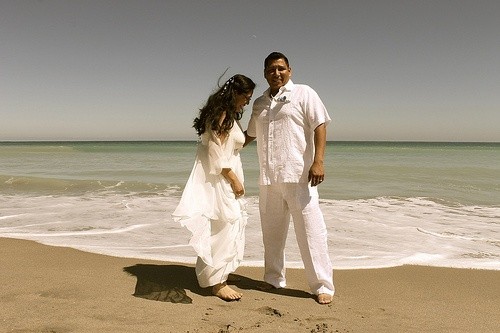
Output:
[320,180,322,181]
[239,194,241,195]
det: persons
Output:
[172,74,255,301]
[242,51,336,304]
